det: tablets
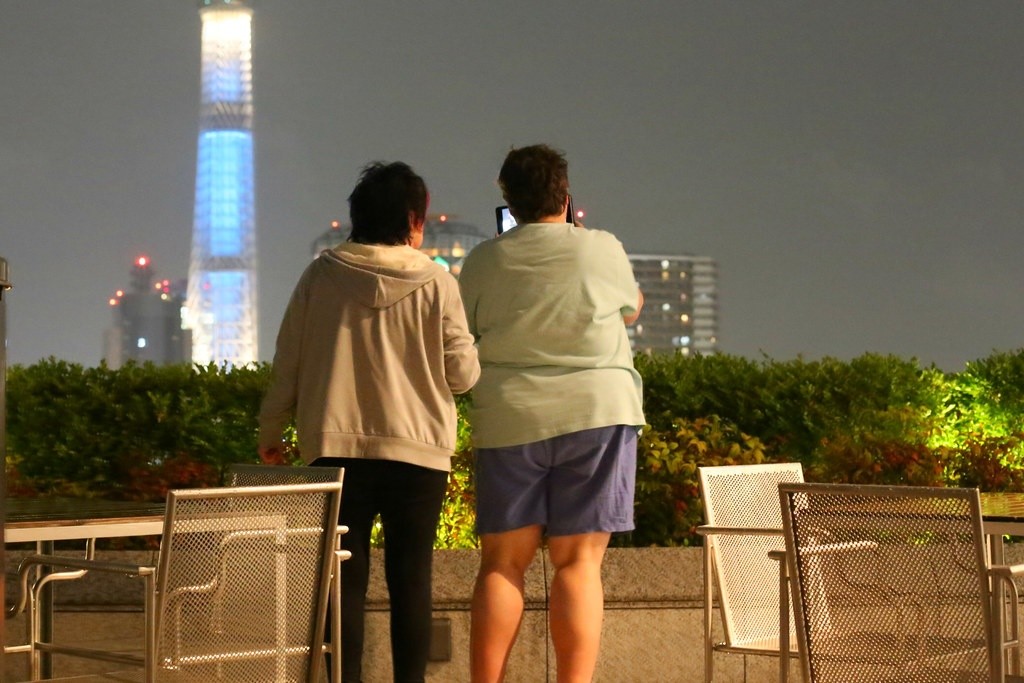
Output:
[495,194,574,236]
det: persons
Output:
[459,143,647,683]
[257,160,481,683]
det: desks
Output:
[799,490,1024,682]
[2,495,286,683]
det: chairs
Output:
[0,460,353,683]
[691,460,1024,683]
